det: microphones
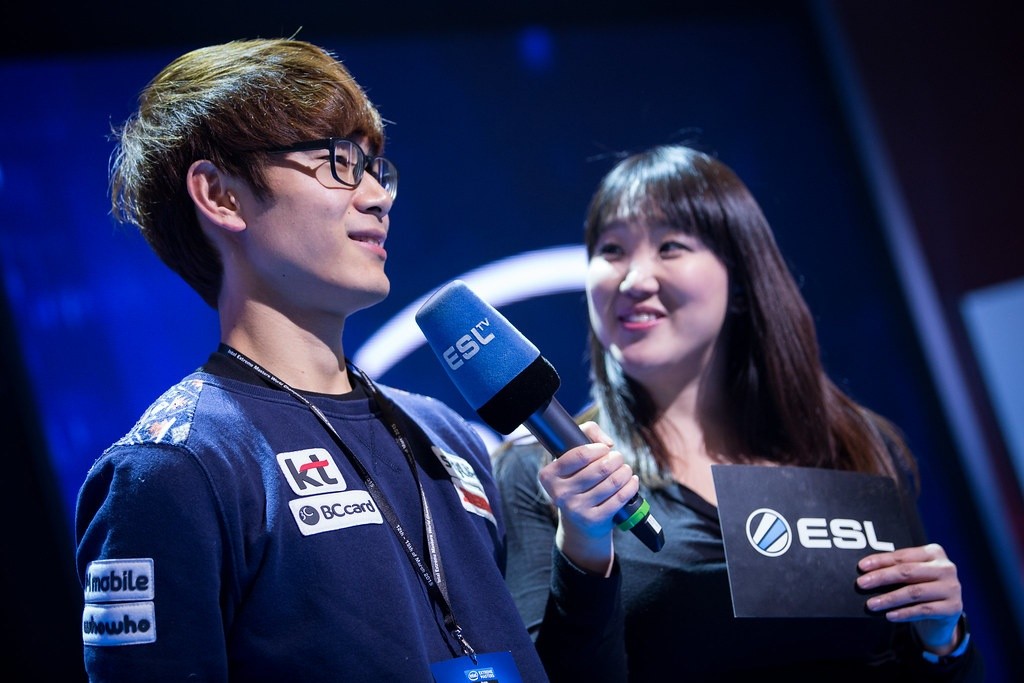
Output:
[414,280,665,552]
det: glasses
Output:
[200,136,398,203]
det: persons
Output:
[496,143,982,683]
[75,41,553,683]
